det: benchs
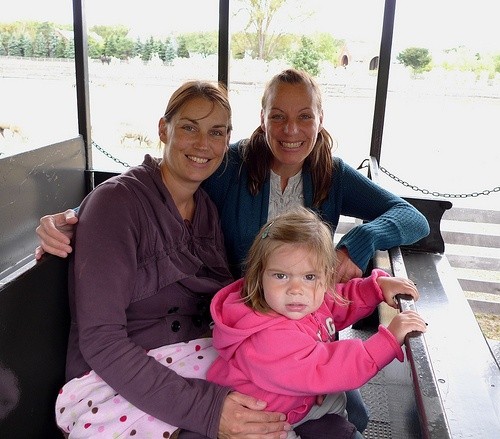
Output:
[0,132,92,439]
[357,156,500,439]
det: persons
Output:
[210,205,428,439]
[37,69,431,439]
[56,80,360,439]
